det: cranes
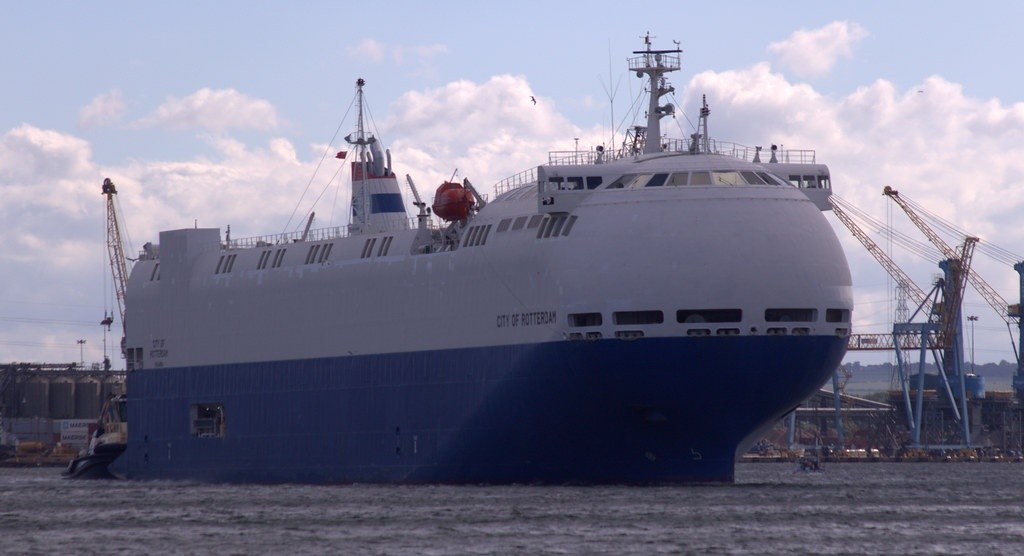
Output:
[823,189,987,396]
[881,185,1024,395]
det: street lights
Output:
[966,314,979,373]
[76,340,86,370]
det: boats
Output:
[59,395,128,480]
[101,29,858,489]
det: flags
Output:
[336,151,347,159]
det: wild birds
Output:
[529,95,537,105]
